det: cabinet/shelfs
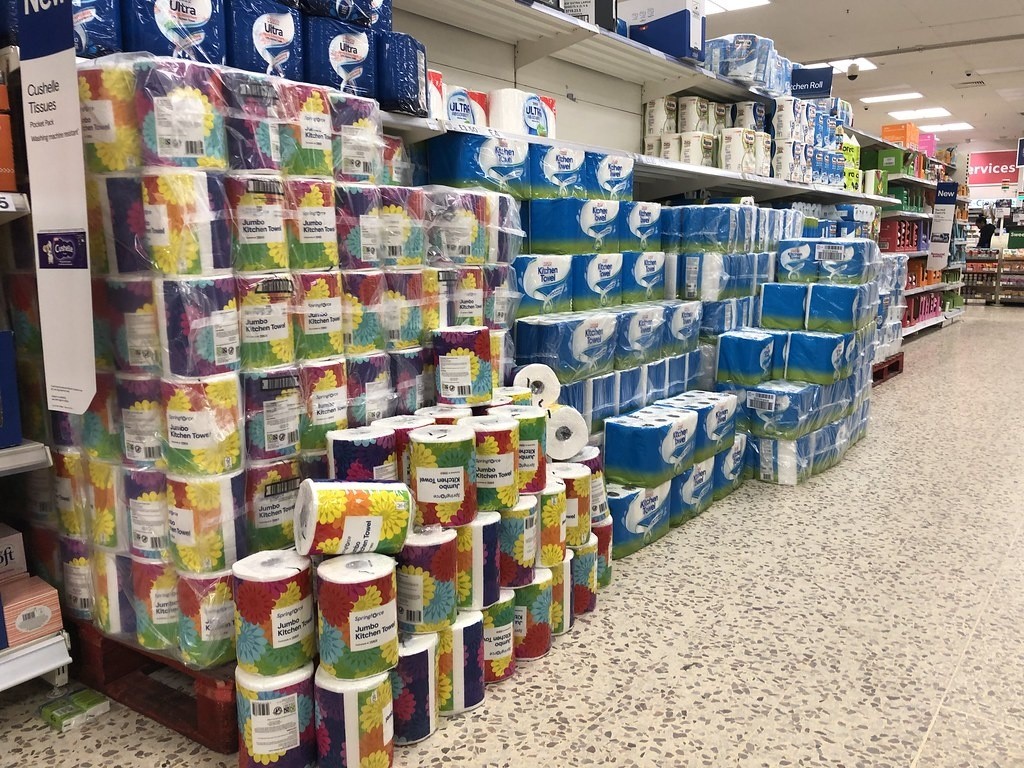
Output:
[598,24,815,201]
[962,247,999,307]
[382,0,598,154]
[881,149,946,337]
[0,42,71,700]
[991,248,1024,308]
[815,124,906,205]
[945,195,972,319]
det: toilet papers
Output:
[661,190,812,299]
[429,563,619,738]
[16,0,429,768]
[641,33,815,184]
[814,97,895,259]
[428,71,748,562]
[743,244,909,489]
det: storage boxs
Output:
[882,122,942,329]
[0,520,66,653]
[611,0,706,65]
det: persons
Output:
[975,216,996,248]
